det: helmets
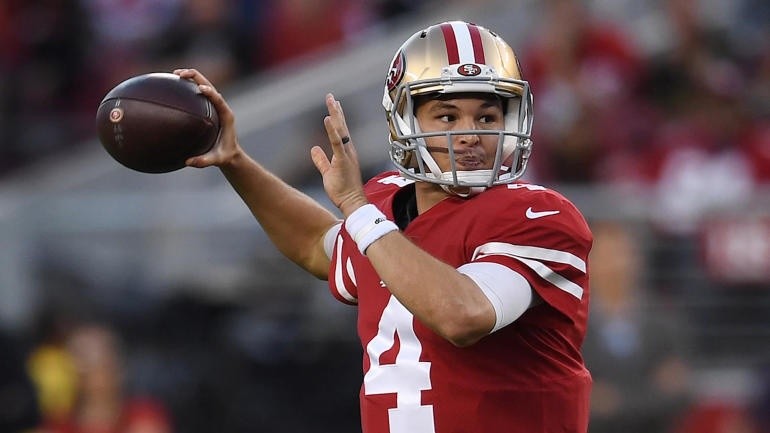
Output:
[382,21,534,198]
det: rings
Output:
[342,135,350,146]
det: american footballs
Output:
[93,70,223,175]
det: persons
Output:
[1,3,388,432]
[518,3,769,433]
[169,19,592,433]
